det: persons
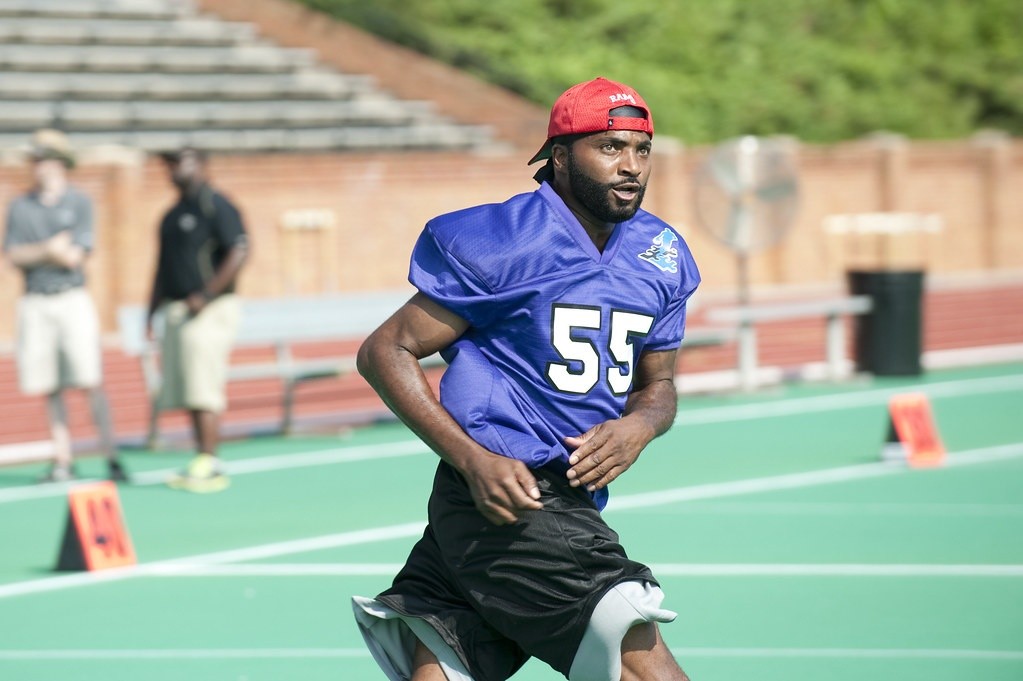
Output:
[4,128,132,481]
[350,75,704,680]
[141,144,249,495]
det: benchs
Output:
[4,0,880,442]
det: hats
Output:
[527,79,654,167]
[26,129,75,168]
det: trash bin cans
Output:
[850,271,925,376]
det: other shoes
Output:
[170,452,229,494]
[45,451,128,487]
